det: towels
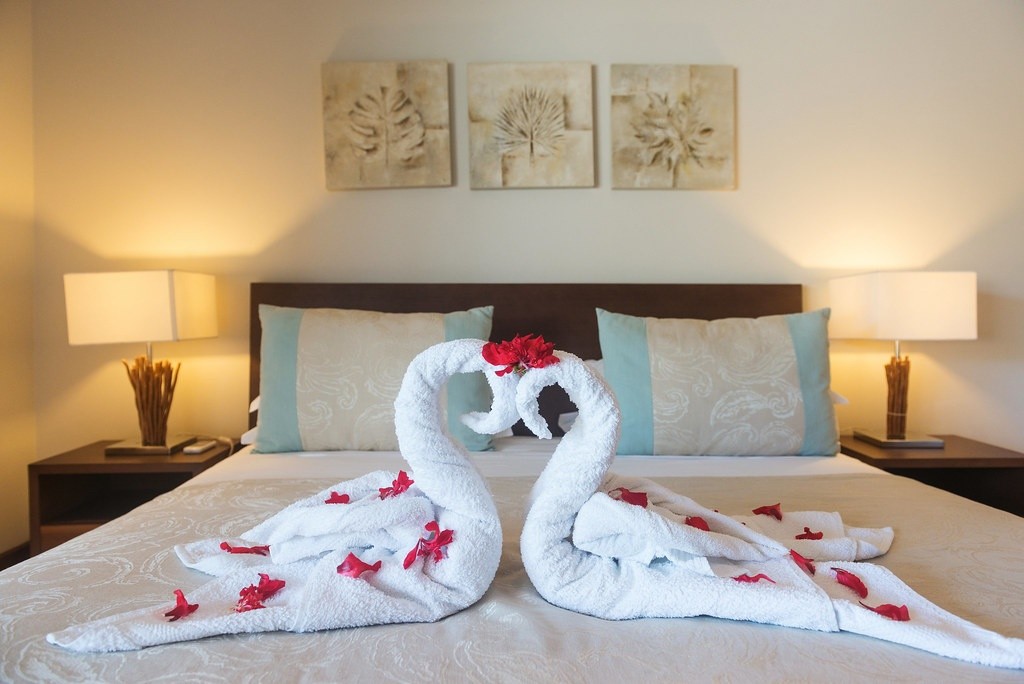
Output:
[41,336,1024,675]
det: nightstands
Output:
[834,430,1024,522]
[28,434,244,566]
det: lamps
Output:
[820,268,981,451]
[61,270,220,453]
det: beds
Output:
[0,433,1023,682]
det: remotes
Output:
[184,440,217,454]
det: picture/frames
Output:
[320,58,455,190]
[467,62,597,190]
[609,62,739,190]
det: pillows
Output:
[249,303,496,455]
[593,308,843,460]
